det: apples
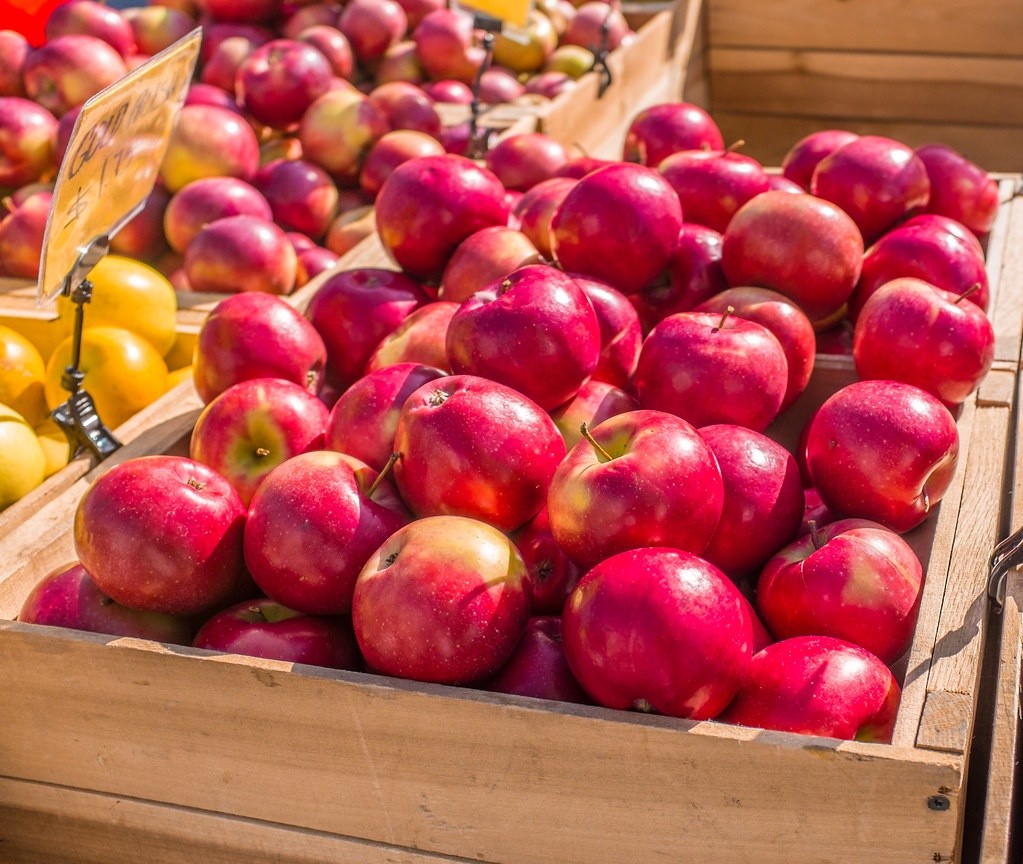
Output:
[0,0,997,740]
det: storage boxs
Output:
[703,0,1023,171]
[0,170,1023,864]
